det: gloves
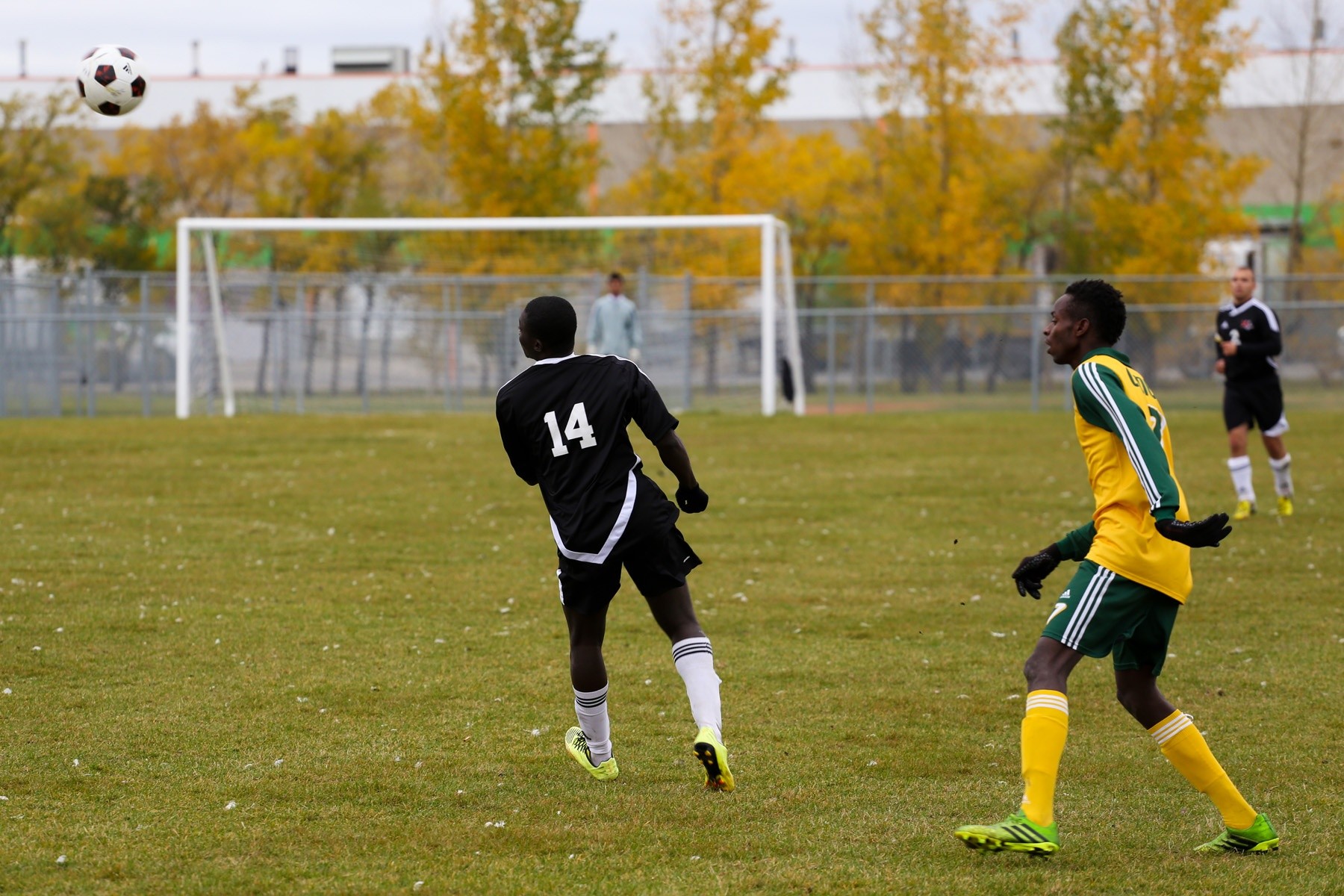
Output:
[1154,512,1232,549]
[1011,543,1062,600]
[675,482,710,514]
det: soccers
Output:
[76,43,151,120]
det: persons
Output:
[953,280,1284,856]
[494,295,737,794]
[1211,266,1299,519]
[586,272,642,369]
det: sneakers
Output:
[1231,499,1259,521]
[1192,812,1280,857]
[692,726,736,793]
[1277,494,1294,517]
[565,725,620,782]
[954,808,1061,861]
